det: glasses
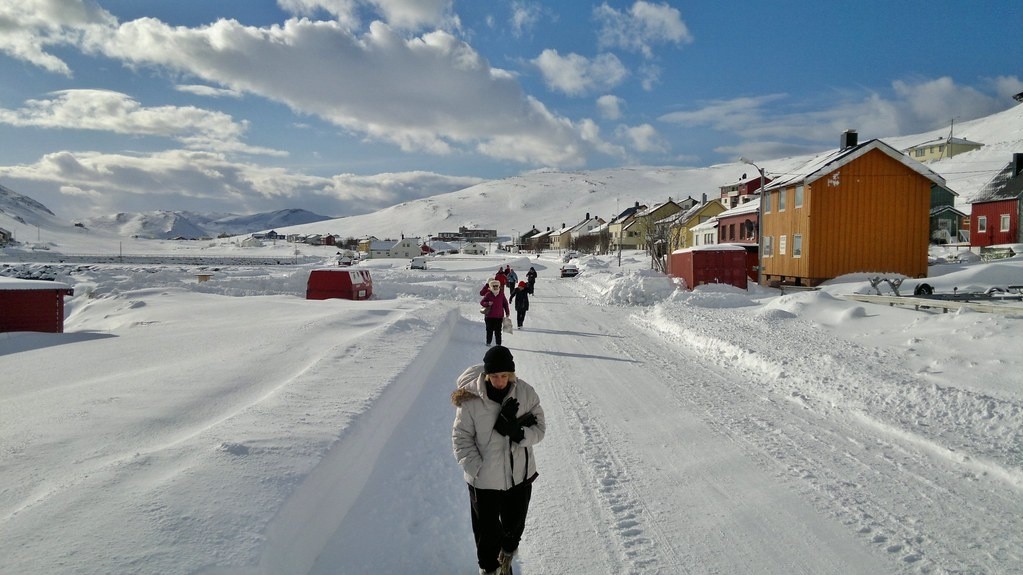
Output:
[493,285,498,287]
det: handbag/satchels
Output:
[502,316,513,332]
[479,294,492,315]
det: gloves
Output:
[493,396,524,443]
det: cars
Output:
[560,265,580,278]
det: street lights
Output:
[737,156,766,284]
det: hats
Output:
[489,280,500,287]
[486,278,494,284]
[518,281,526,288]
[483,345,516,375]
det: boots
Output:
[497,550,514,575]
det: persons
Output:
[509,280,530,329]
[450,345,546,575]
[480,281,510,347]
[526,266,538,297]
[494,264,518,296]
[480,277,495,296]
